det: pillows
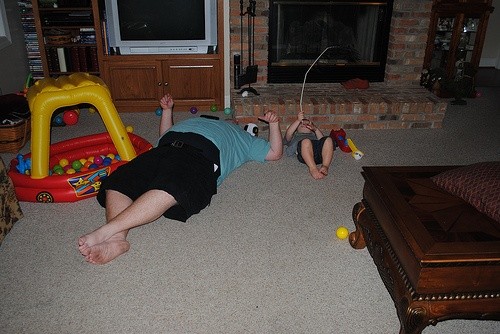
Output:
[430,162,500,223]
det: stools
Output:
[27,72,138,178]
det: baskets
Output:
[0,116,31,154]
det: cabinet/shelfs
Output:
[423,0,495,88]
[32,0,224,112]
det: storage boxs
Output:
[0,93,31,154]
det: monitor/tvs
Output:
[104,0,217,54]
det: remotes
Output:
[200,115,219,120]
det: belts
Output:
[167,140,198,158]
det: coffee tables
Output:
[349,165,500,334]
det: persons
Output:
[78,92,284,264]
[287,111,334,180]
